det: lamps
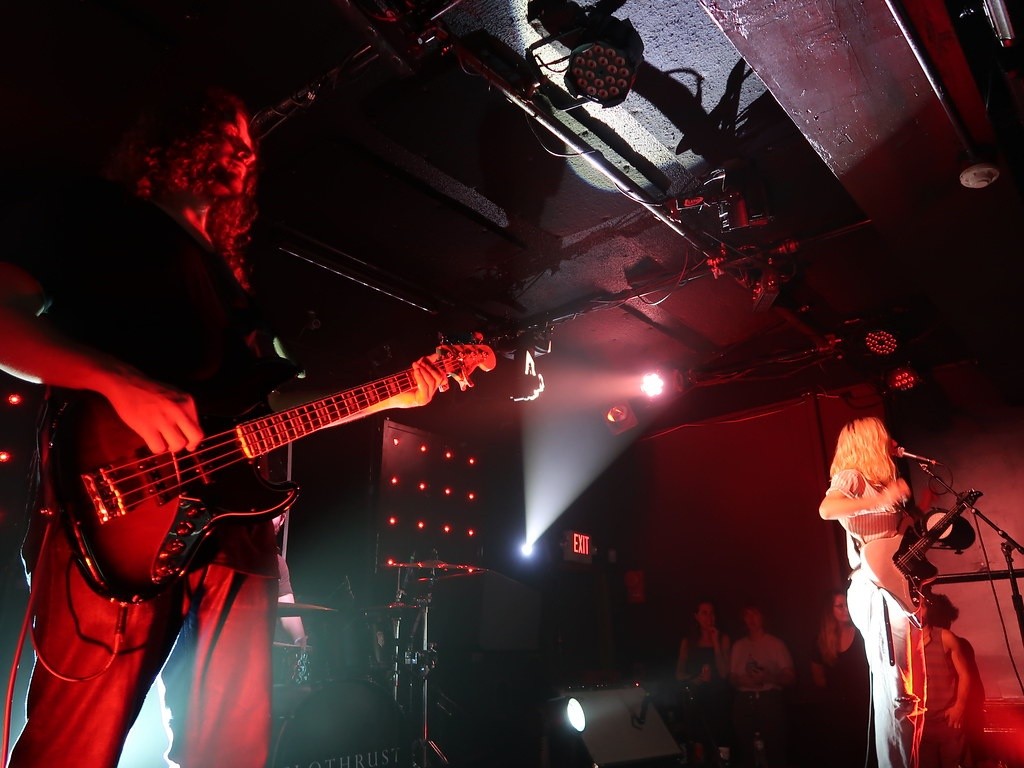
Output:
[563,17,643,110]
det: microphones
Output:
[894,446,942,466]
[638,701,648,725]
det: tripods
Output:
[411,591,450,768]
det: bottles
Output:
[743,654,758,673]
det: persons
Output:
[915,590,969,768]
[818,416,928,768]
[0,81,449,768]
[811,592,869,692]
[676,598,730,767]
[729,600,794,768]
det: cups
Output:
[718,747,730,767]
[753,732,767,768]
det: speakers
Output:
[982,698,1024,768]
[560,682,683,768]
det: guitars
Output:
[862,488,984,617]
[36,329,499,605]
